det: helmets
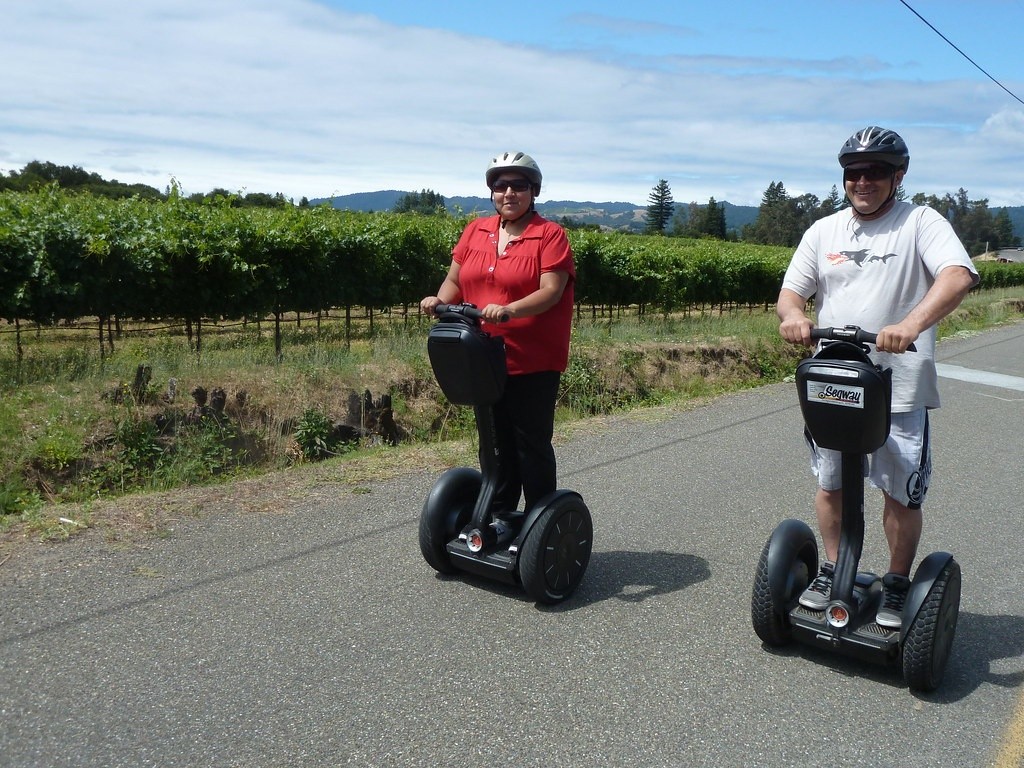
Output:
[486,151,542,191]
[838,127,910,176]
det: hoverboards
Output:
[752,323,964,696]
[418,304,596,606]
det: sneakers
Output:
[798,560,836,610]
[875,572,910,627]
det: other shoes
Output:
[458,518,491,540]
[508,534,520,552]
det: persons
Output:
[778,127,980,630]
[421,151,576,556]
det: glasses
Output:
[491,179,533,192]
[844,163,896,181]
